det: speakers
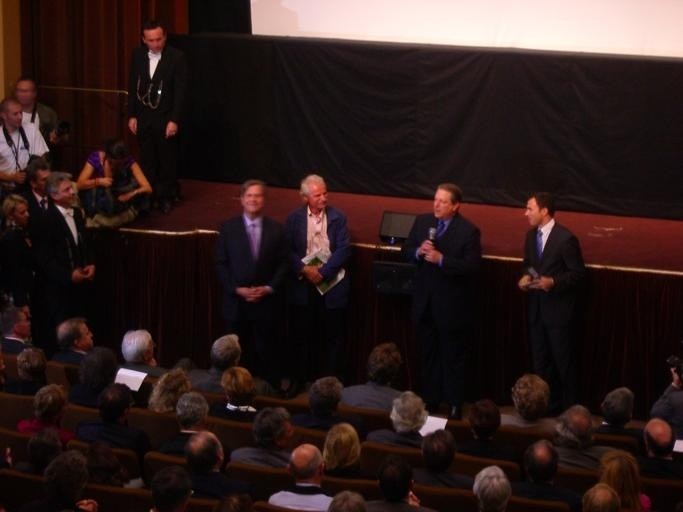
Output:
[378,210,417,241]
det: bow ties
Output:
[61,208,74,218]
[147,52,162,61]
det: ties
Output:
[249,223,258,260]
[435,221,444,240]
[40,199,46,217]
[536,229,543,260]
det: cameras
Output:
[666,355,683,376]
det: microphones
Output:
[428,227,438,246]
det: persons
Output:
[128,22,187,213]
[280,172,353,385]
[405,176,480,416]
[211,178,293,370]
[1,292,681,512]
[514,189,588,401]
[0,78,153,306]
